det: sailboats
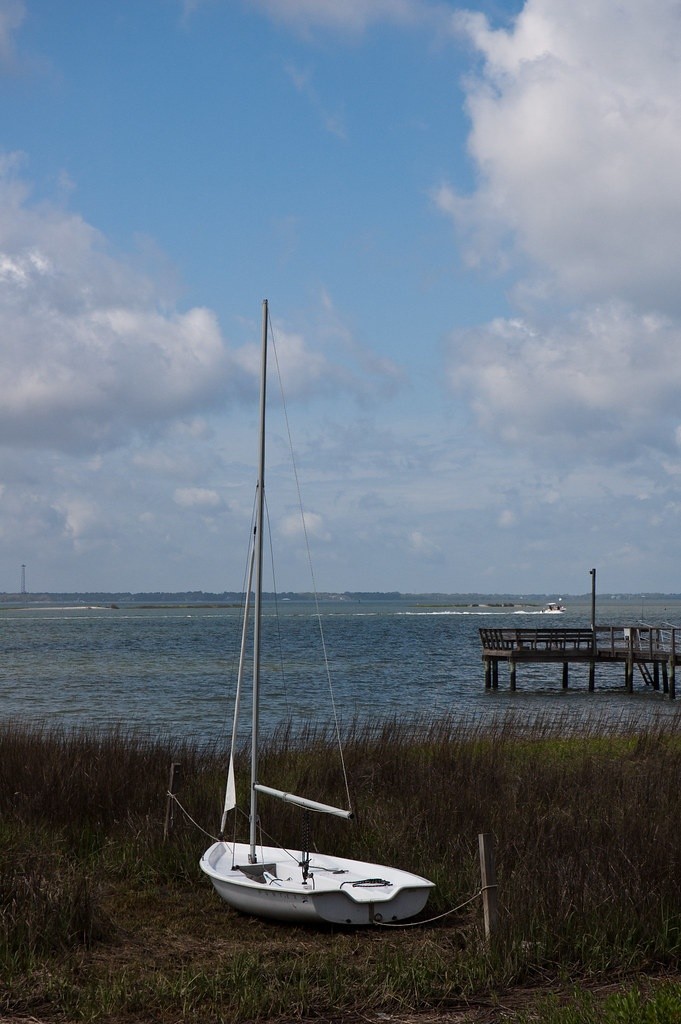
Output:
[200,298,436,926]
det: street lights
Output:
[641,596,645,622]
[589,568,597,653]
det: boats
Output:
[540,603,563,614]
